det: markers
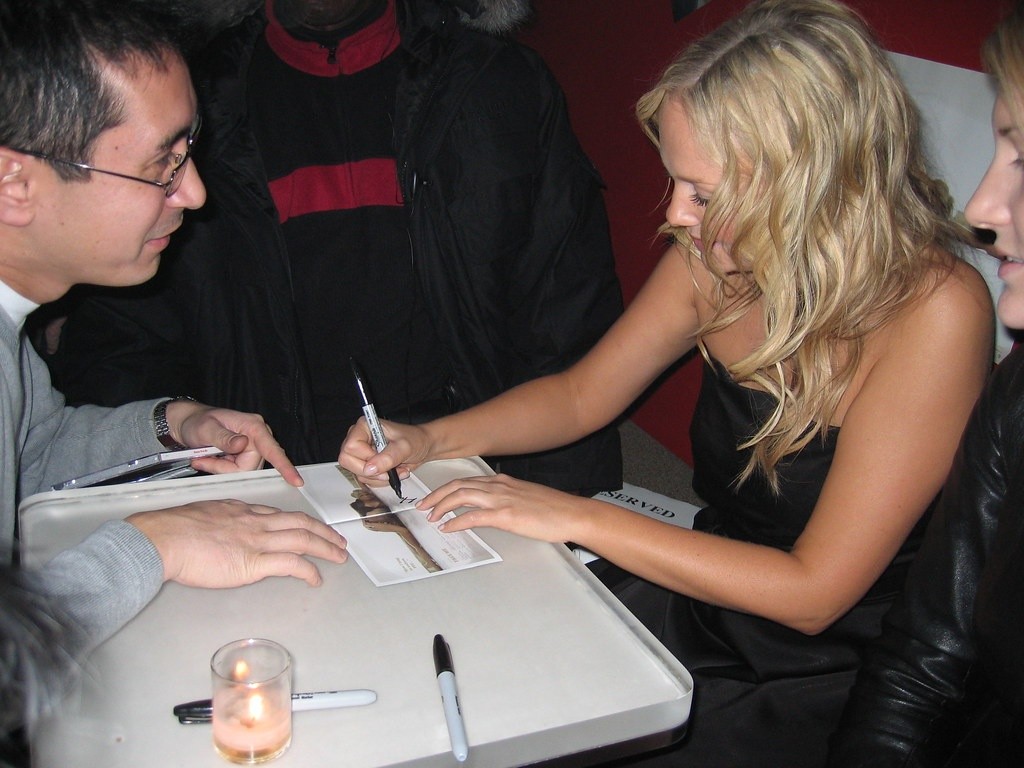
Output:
[433,633,467,762]
[173,690,378,725]
[349,356,401,498]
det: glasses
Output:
[0,114,203,197]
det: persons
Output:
[827,0,1024,767]
[0,0,348,768]
[336,0,997,767]
[47,0,624,496]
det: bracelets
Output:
[154,396,201,450]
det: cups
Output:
[211,637,294,765]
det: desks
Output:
[20,454,694,768]
